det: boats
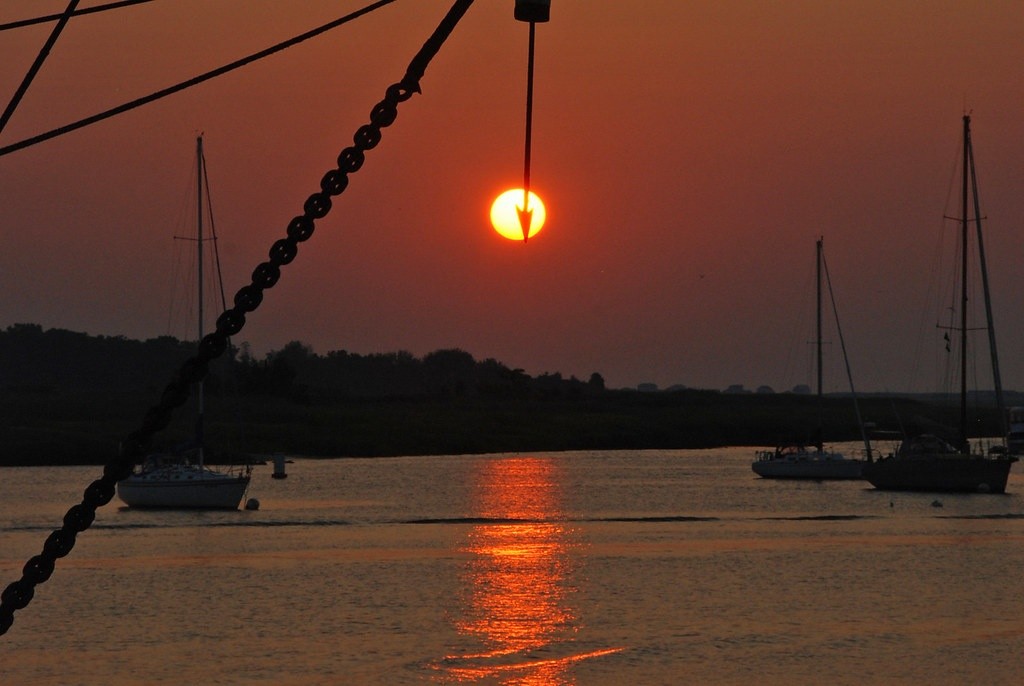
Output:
[1006,404,1024,454]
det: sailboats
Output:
[753,235,877,481]
[111,131,260,512]
[863,108,1019,496]
